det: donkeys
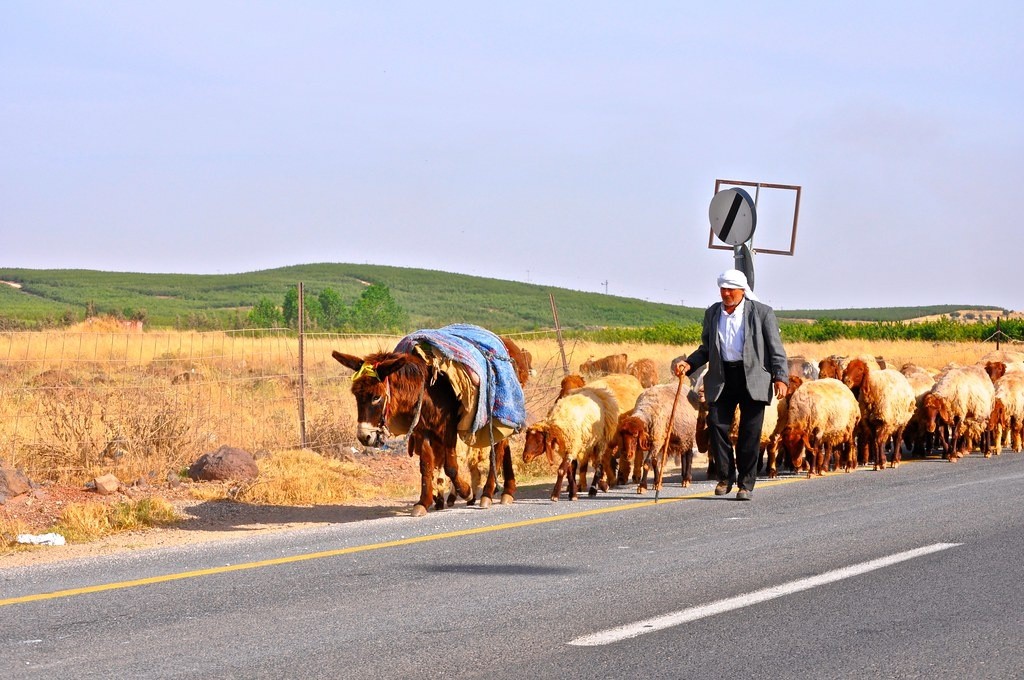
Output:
[329,336,537,517]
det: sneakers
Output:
[715,478,735,495]
[736,487,751,500]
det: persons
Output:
[674,269,789,500]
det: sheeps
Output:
[523,353,1023,503]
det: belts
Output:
[722,360,743,368]
